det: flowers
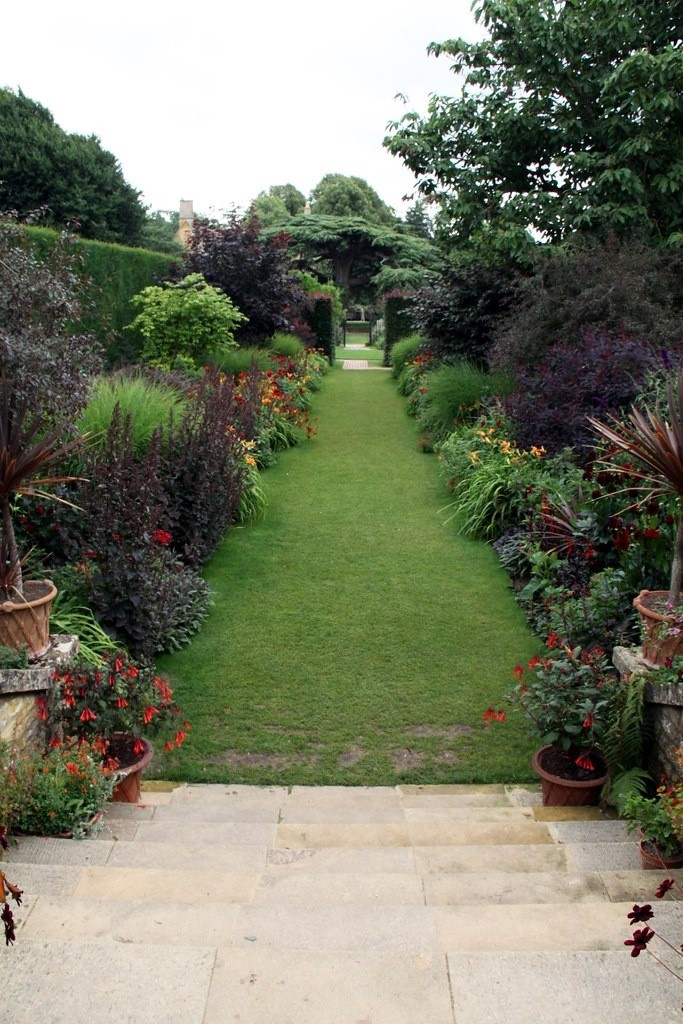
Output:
[0,640,189,841]
[620,745,683,857]
[481,630,627,772]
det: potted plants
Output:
[582,368,683,669]
[0,364,107,660]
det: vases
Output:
[58,810,101,838]
[100,732,151,803]
[533,745,609,807]
[638,826,683,870]
